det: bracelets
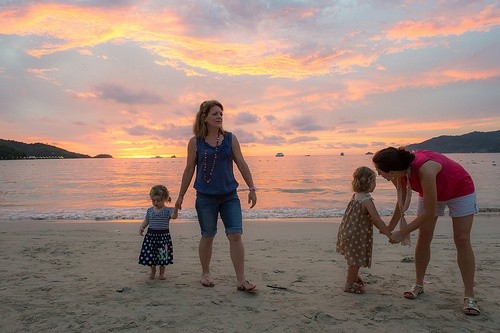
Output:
[250,187,257,191]
[174,211,179,213]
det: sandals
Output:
[404,284,424,299]
[237,278,256,291]
[463,296,480,315]
[355,277,365,287]
[344,284,365,294]
[199,276,215,287]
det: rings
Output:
[396,237,399,240]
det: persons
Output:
[372,146,481,316]
[175,100,258,292]
[139,184,183,280]
[336,166,394,295]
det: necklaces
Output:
[204,130,221,184]
